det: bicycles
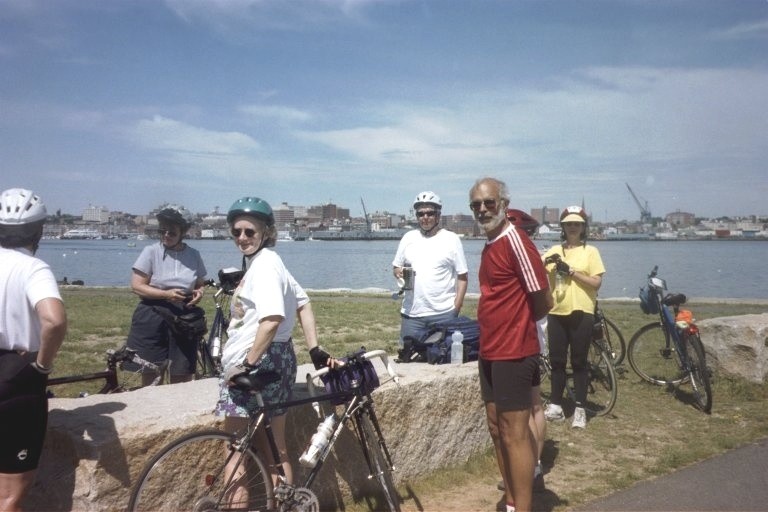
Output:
[194,266,244,378]
[626,262,713,413]
[564,291,626,415]
[46,347,169,398]
[123,347,404,512]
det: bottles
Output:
[210,336,220,358]
[555,271,565,291]
[396,262,414,290]
[451,330,463,365]
[298,412,336,468]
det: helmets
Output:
[0,188,48,227]
[561,207,588,220]
[226,196,275,226]
[507,208,537,236]
[157,204,192,231]
[414,191,442,210]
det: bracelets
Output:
[36,360,52,371]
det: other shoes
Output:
[498,466,544,490]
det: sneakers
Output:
[544,404,565,423]
[572,405,587,429]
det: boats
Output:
[654,231,677,241]
[62,229,103,240]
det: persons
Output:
[467,178,554,512]
[214,195,342,512]
[541,205,606,431]
[391,192,469,348]
[130,204,207,386]
[0,188,67,512]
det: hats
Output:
[560,214,586,223]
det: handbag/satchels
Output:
[172,305,208,340]
[639,285,659,314]
[321,356,380,405]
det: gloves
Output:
[556,259,575,277]
[545,254,561,263]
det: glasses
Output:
[230,227,265,238]
[469,197,504,211]
[417,210,439,217]
[156,228,177,236]
